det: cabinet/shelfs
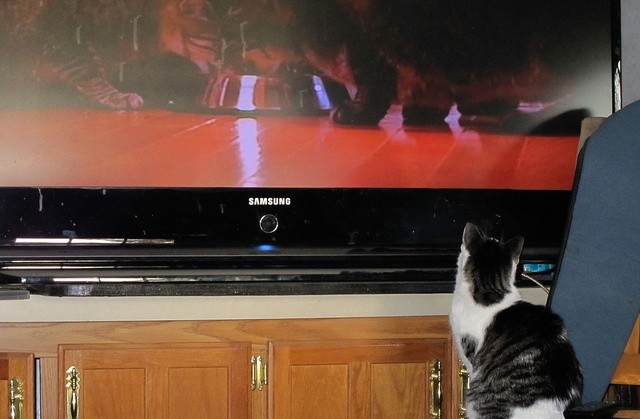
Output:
[0,322,256,418]
[255,315,469,419]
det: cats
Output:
[448,220,584,419]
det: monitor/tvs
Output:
[0,0,623,294]
